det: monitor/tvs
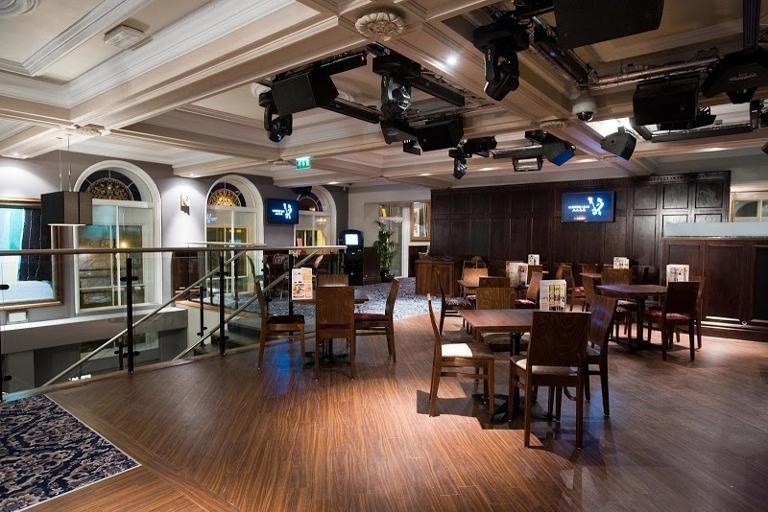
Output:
[560,190,616,223]
[265,197,300,224]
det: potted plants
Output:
[374,218,395,283]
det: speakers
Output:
[544,143,575,166]
[415,119,462,151]
[600,132,637,158]
[271,69,339,116]
[632,77,698,125]
[555,0,664,48]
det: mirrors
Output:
[0,196,65,312]
[410,200,430,242]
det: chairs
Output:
[544,293,620,416]
[315,287,357,380]
[316,273,349,288]
[508,308,593,448]
[254,280,305,367]
[426,292,495,418]
[354,278,400,363]
[438,261,706,362]
[475,285,530,396]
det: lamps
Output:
[260,99,298,143]
[479,43,527,105]
[597,66,732,164]
[375,73,416,119]
[401,116,581,177]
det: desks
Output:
[293,290,369,369]
[458,305,537,423]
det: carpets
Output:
[191,276,442,325]
[0,393,142,512]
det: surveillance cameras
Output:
[572,92,598,122]
